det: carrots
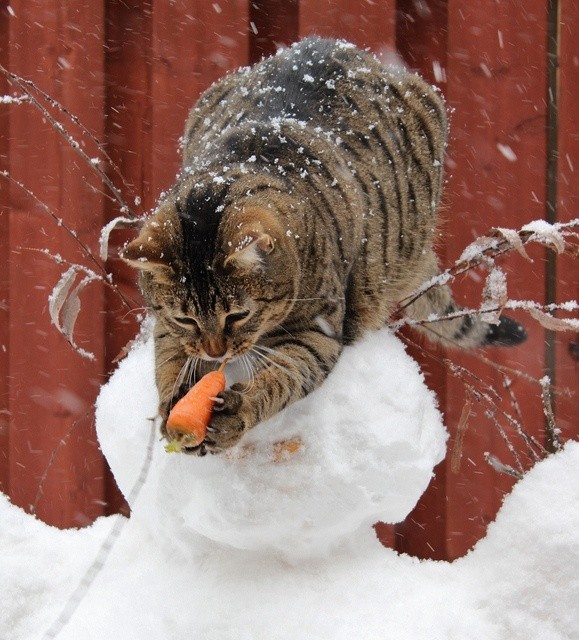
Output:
[168,360,227,454]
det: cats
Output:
[116,32,530,460]
